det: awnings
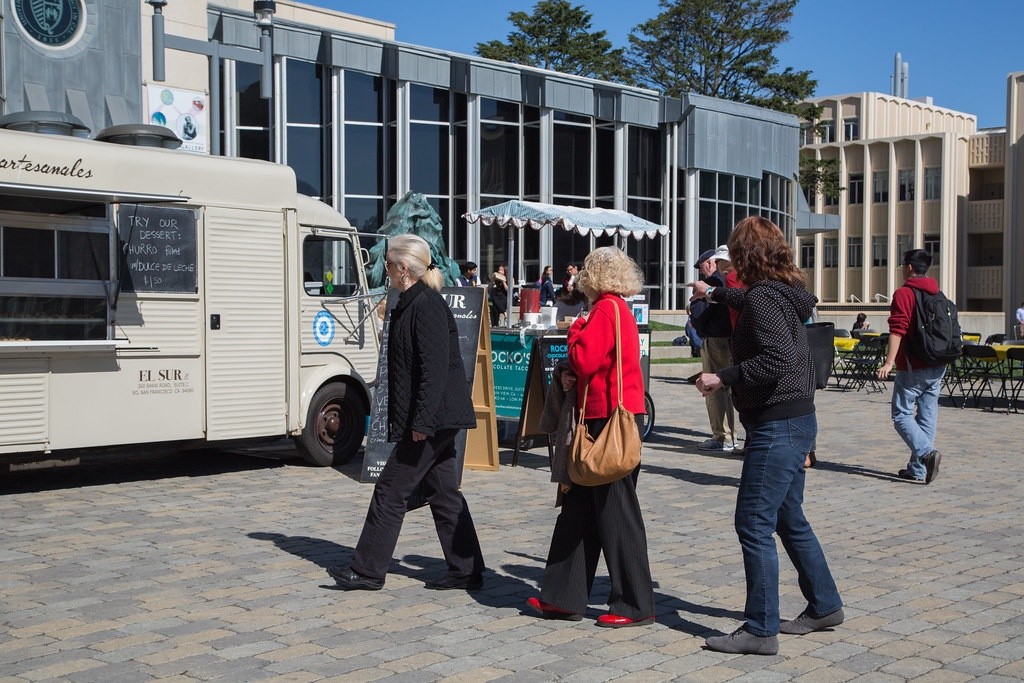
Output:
[0,183,191,309]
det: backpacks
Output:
[910,286,963,364]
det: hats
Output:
[710,245,731,261]
[694,249,716,268]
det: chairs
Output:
[821,321,1024,416]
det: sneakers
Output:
[697,439,738,452]
[779,609,844,634]
[426,573,484,591]
[328,565,386,591]
[704,629,779,655]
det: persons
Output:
[328,237,487,590]
[524,245,659,628]
[464,244,817,469]
[877,249,946,484]
[684,215,845,655]
[1015,303,1024,340]
[852,313,869,331]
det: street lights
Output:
[146,0,275,155]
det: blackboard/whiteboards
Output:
[115,205,197,295]
[511,337,570,477]
[358,285,500,483]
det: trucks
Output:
[0,110,391,467]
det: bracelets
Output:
[704,285,718,304]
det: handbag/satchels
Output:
[569,406,641,486]
[805,321,835,388]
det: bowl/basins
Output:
[555,322,570,329]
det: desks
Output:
[834,336,860,361]
[977,341,1024,360]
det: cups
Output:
[523,313,543,325]
[564,316,572,323]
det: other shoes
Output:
[898,469,921,481]
[925,450,943,484]
[596,614,656,628]
[527,597,583,621]
[803,452,816,468]
